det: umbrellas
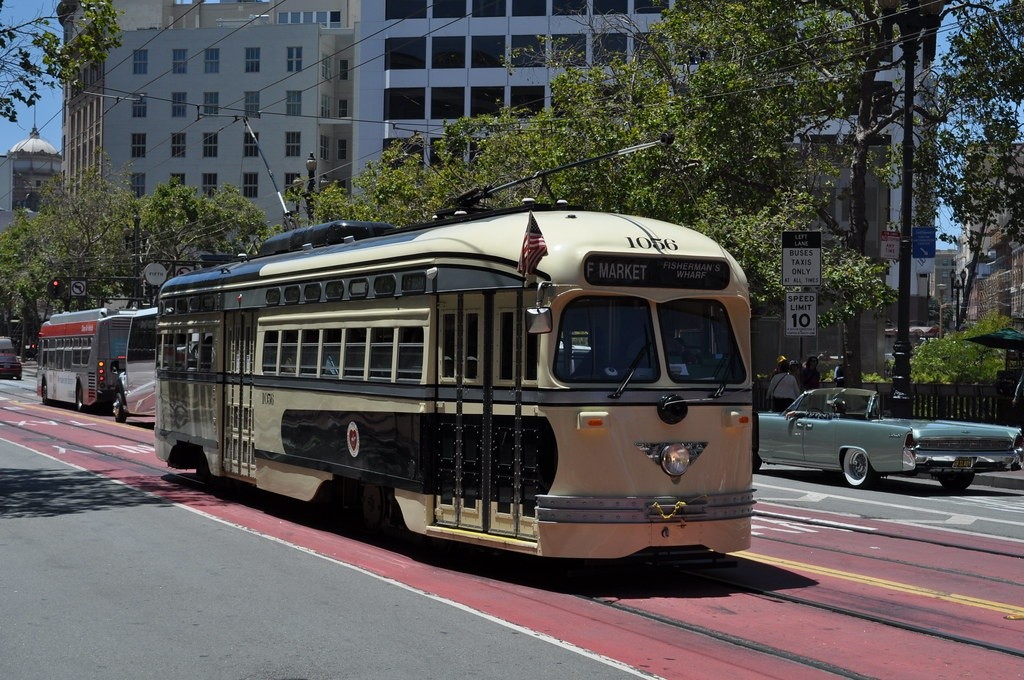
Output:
[961,327,1024,372]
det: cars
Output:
[0,335,22,380]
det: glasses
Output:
[811,360,817,363]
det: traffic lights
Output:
[47,277,66,300]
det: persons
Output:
[765,354,821,412]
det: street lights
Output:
[305,153,319,226]
[292,170,304,215]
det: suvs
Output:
[749,389,1024,493]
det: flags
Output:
[517,213,548,276]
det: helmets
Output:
[789,360,801,366]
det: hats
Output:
[776,356,786,364]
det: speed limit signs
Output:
[785,291,817,334]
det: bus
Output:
[36,305,136,424]
[153,198,762,590]
